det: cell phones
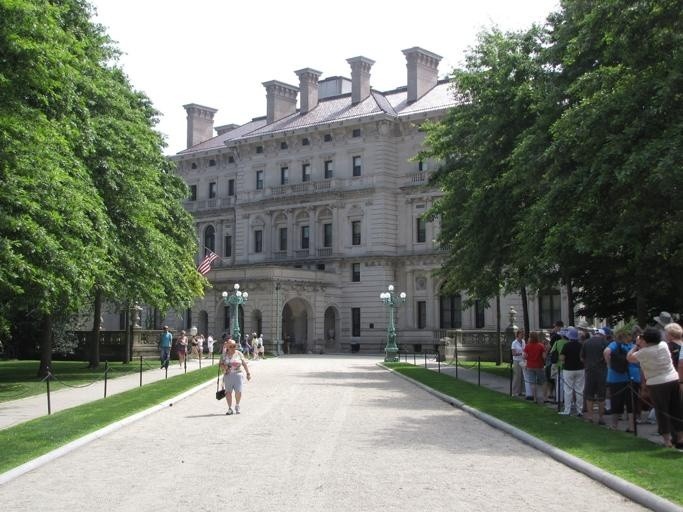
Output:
[640,334,645,340]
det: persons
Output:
[511,311,682,450]
[218,339,252,415]
[158,326,291,369]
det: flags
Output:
[197,249,218,275]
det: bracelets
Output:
[247,373,250,375]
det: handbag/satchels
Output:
[611,349,628,373]
[551,350,559,363]
[216,390,226,400]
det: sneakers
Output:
[226,408,233,415]
[511,391,594,422]
[235,405,241,414]
[598,407,657,433]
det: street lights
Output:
[221,283,247,352]
[378,284,407,362]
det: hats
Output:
[653,312,673,327]
[556,321,611,340]
